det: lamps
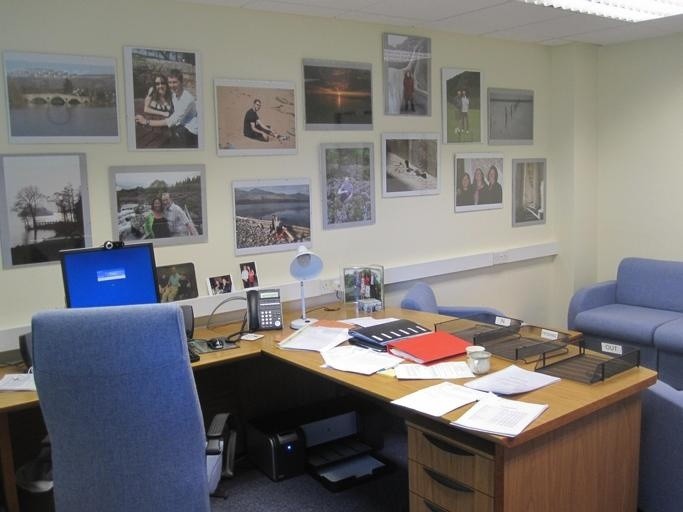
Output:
[289,246,324,330]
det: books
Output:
[345,319,468,373]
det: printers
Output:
[249,406,389,493]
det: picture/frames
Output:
[109,164,208,248]
[320,142,376,232]
[207,274,236,296]
[123,48,204,150]
[340,264,385,312]
[156,262,199,303]
[3,51,121,144]
[240,261,259,288]
[302,58,374,130]
[232,178,314,256]
[382,32,548,227]
[212,79,298,156]
[0,153,93,270]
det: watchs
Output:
[145,119,151,125]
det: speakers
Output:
[181,305,194,337]
[19,332,33,368]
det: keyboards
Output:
[188,347,200,362]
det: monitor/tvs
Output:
[59,242,160,309]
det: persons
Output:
[143,75,173,117]
[244,99,289,143]
[269,215,288,238]
[453,89,462,134]
[457,166,500,205]
[214,279,222,292]
[353,269,377,303]
[247,265,255,286]
[241,265,249,288]
[133,68,198,143]
[132,192,197,239]
[337,176,354,203]
[158,266,194,301]
[403,70,415,112]
[462,90,470,134]
[221,278,231,292]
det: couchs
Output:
[643,376,683,510]
[401,282,503,325]
[567,258,683,389]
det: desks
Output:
[210,301,662,510]
[0,328,261,511]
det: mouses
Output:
[207,337,226,350]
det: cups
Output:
[469,351,491,375]
[465,345,486,366]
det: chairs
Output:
[30,304,239,511]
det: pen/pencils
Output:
[300,281,305,320]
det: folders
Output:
[387,331,473,366]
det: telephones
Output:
[246,287,283,332]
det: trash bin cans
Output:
[16,460,54,512]
